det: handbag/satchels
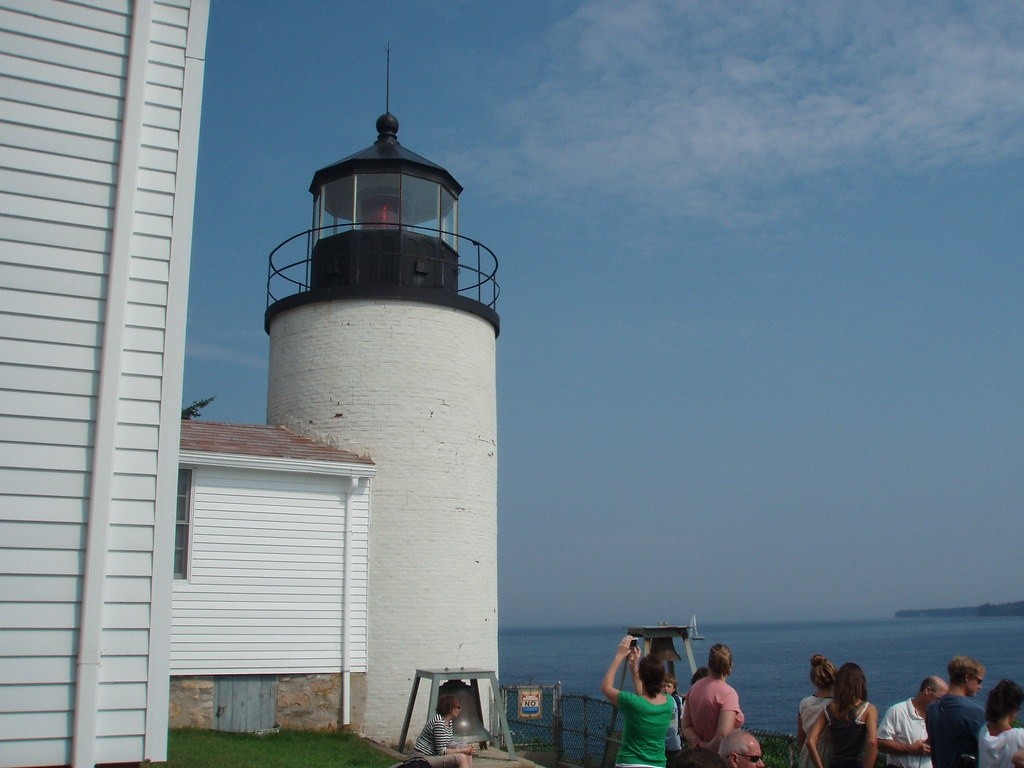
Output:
[960,752,977,768]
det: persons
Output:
[661,674,686,768]
[680,644,764,768]
[978,679,1024,768]
[876,675,950,768]
[925,657,987,768]
[798,655,878,768]
[601,635,674,768]
[412,693,475,768]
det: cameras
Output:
[629,637,639,649]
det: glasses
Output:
[726,754,763,762]
[453,705,462,709]
[969,673,983,684]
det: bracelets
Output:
[458,750,459,752]
